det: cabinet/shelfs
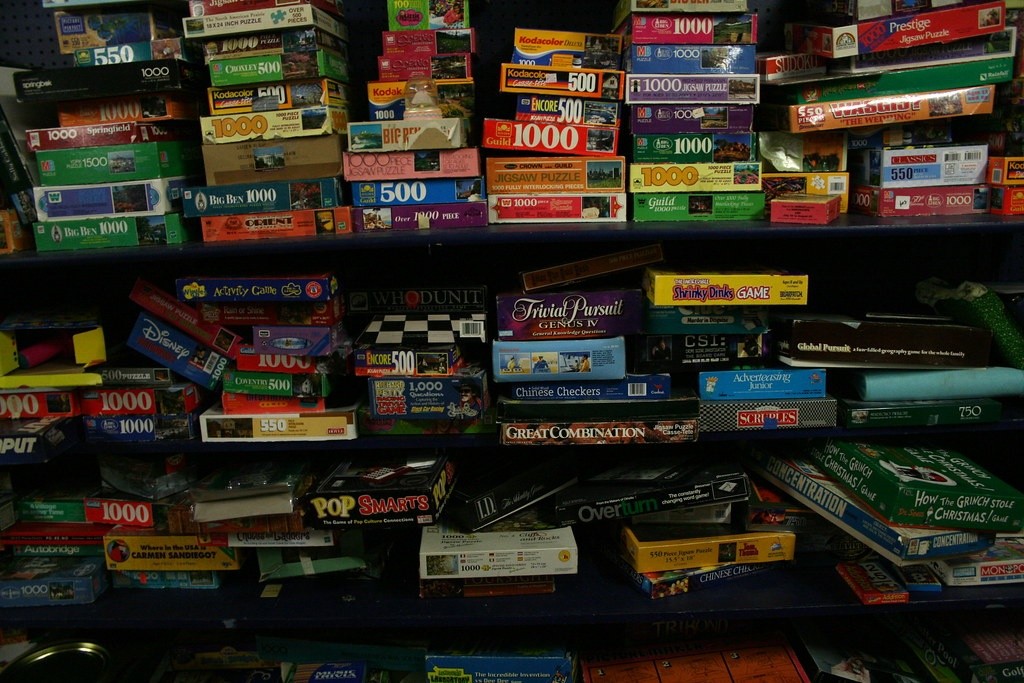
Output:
[0,0,1024,683]
